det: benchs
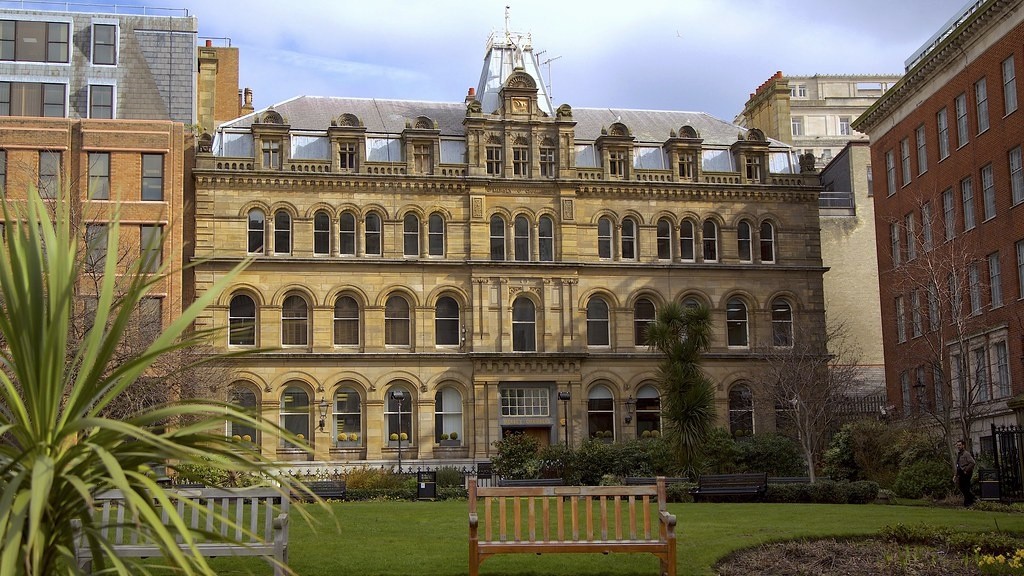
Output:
[468,476,677,576]
[621,476,691,501]
[289,480,348,503]
[498,477,566,503]
[173,483,206,503]
[70,478,289,576]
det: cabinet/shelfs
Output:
[691,472,768,502]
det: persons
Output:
[952,440,977,510]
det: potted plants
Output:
[734,429,752,442]
[641,430,660,442]
[291,434,307,448]
[336,433,357,447]
[388,433,409,447]
[229,434,252,448]
[440,432,460,446]
[596,431,613,444]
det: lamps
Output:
[912,378,924,404]
[318,396,329,432]
[624,395,635,424]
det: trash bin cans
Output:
[979,468,1000,501]
[417,471,436,501]
